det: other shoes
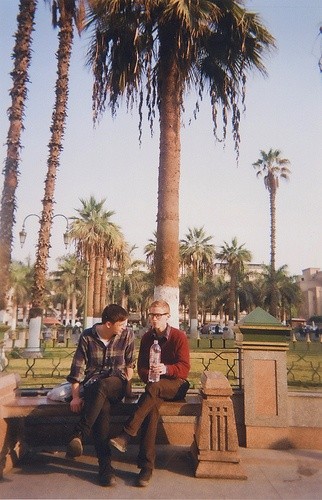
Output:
[99,473,118,486]
[135,465,153,487]
[65,437,83,458]
[109,435,128,453]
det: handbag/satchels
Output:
[46,377,85,403]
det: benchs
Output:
[0,371,248,482]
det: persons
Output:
[67,303,137,486]
[215,324,219,333]
[108,299,191,488]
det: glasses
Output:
[147,312,170,319]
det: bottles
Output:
[148,340,160,382]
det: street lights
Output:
[19,214,70,250]
